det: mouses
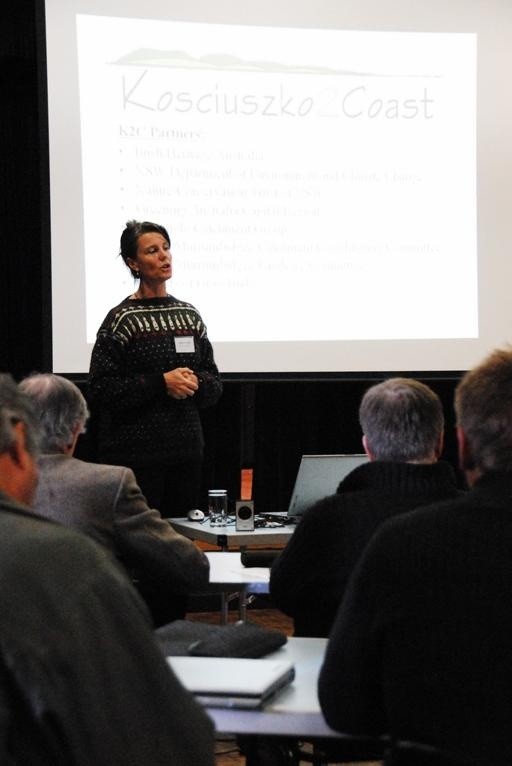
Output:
[187,509,205,522]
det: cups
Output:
[208,488,228,527]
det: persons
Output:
[269,377,466,638]
[86,221,222,515]
[0,375,216,764]
[318,348,512,765]
[18,370,210,628]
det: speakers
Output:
[235,500,255,530]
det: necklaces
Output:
[134,291,171,299]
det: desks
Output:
[189,547,289,627]
[162,629,406,765]
[164,512,298,625]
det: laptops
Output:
[257,453,371,523]
[164,654,295,712]
